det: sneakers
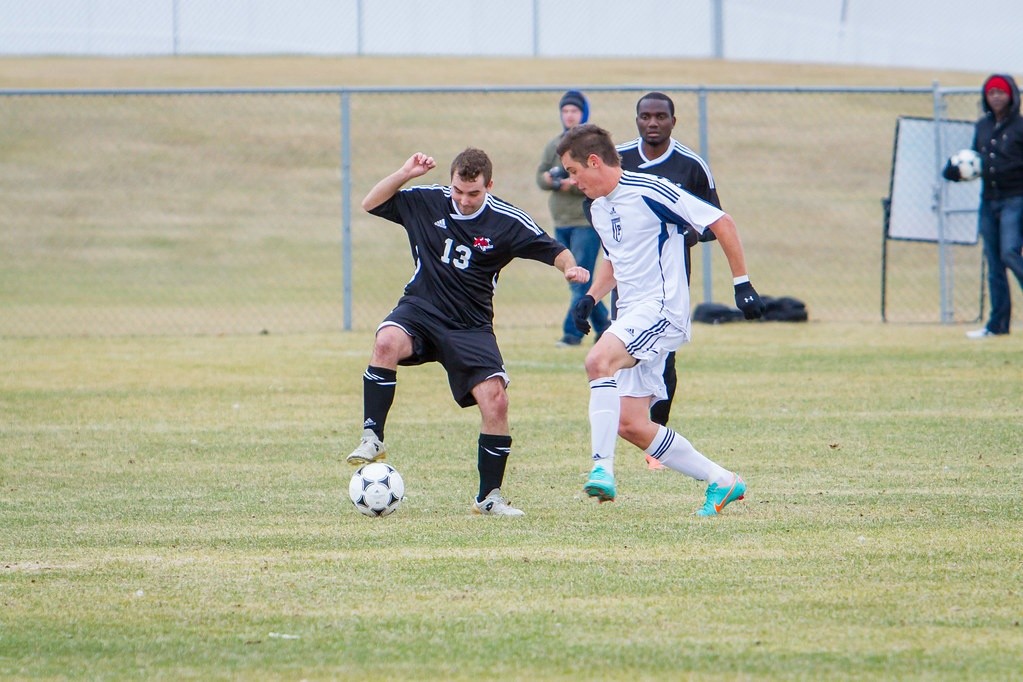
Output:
[695,471,746,516]
[583,465,616,504]
[346,429,386,465]
[471,487,526,517]
[966,328,996,338]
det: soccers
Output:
[950,148,983,181]
[348,462,406,519]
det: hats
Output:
[985,76,1012,96]
[560,92,584,112]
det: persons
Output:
[536,91,613,347]
[556,123,766,516]
[941,74,1023,340]
[581,92,723,471]
[344,144,590,515]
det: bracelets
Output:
[552,178,560,191]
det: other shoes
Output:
[645,454,664,471]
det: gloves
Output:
[574,294,595,335]
[942,159,961,183]
[734,281,766,320]
